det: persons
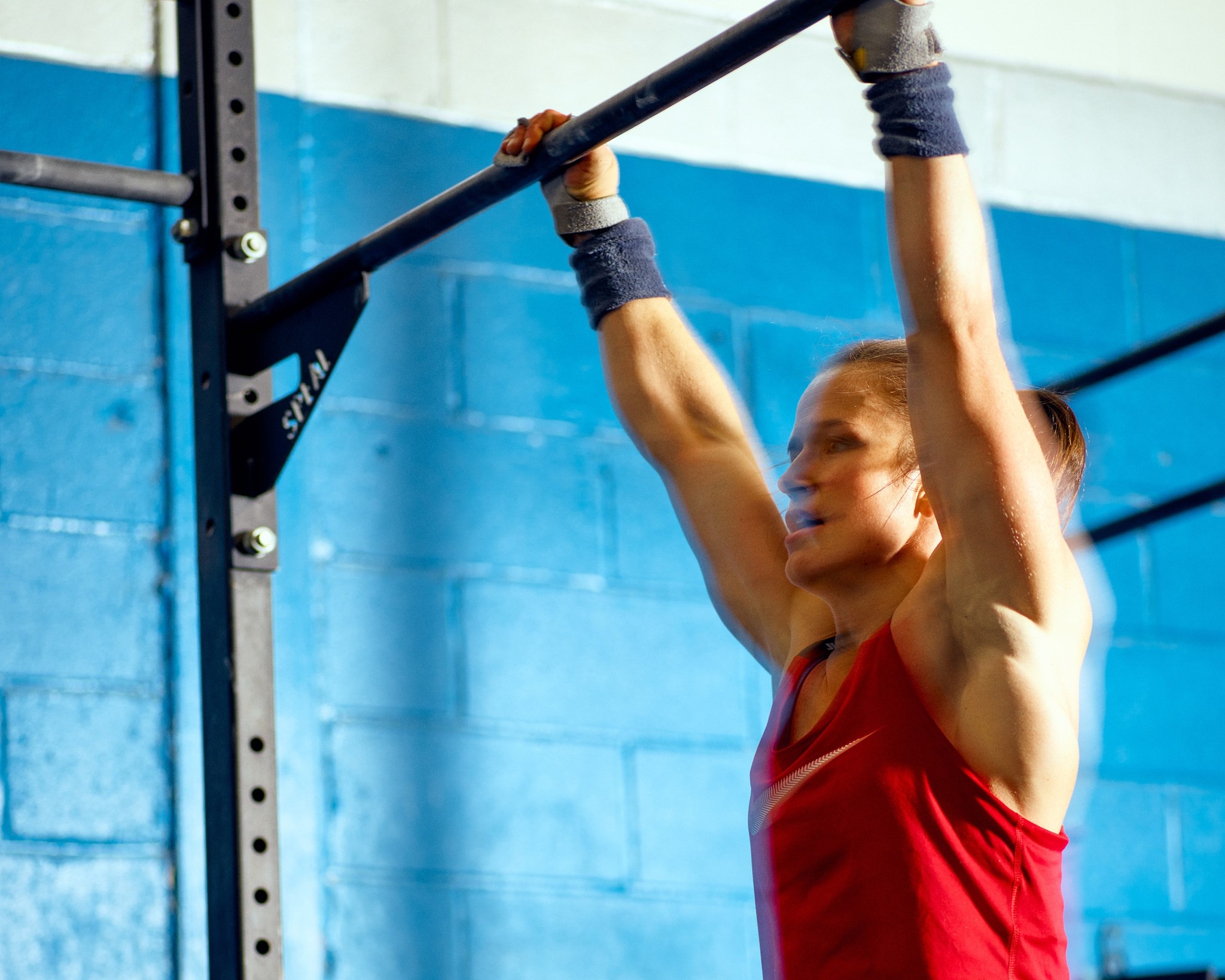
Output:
[500,0,1091,980]
